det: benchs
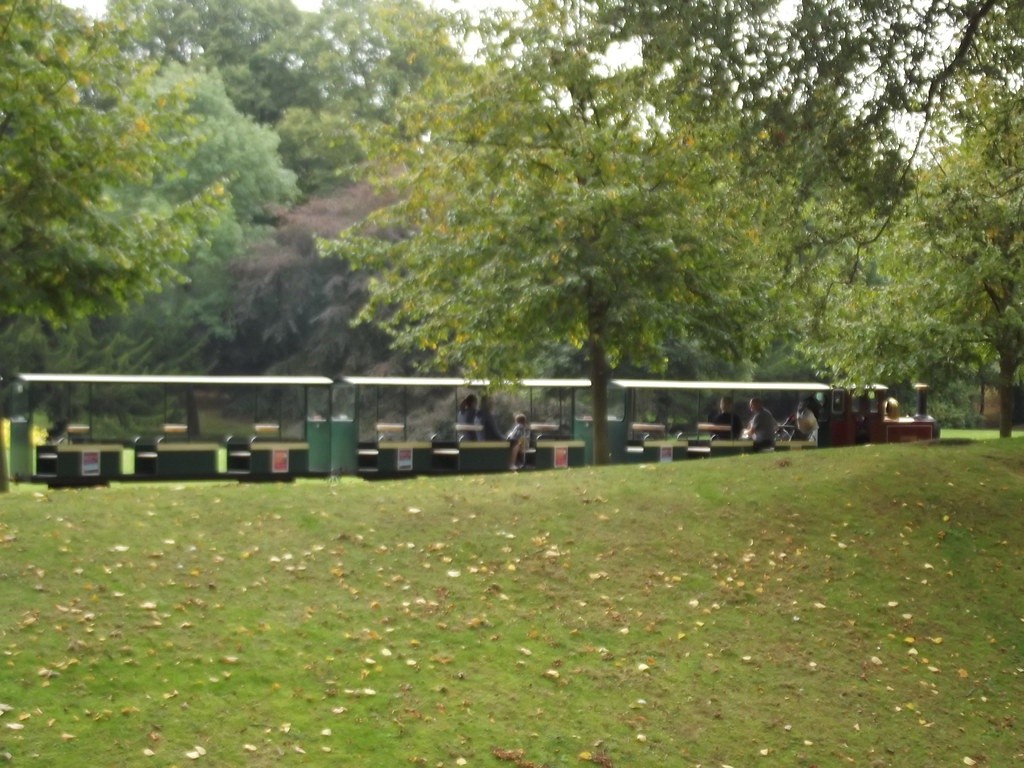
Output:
[57,422,734,471]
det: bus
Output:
[10,374,938,486]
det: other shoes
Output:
[510,464,519,470]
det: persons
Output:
[792,401,818,441]
[744,398,776,453]
[507,414,530,471]
[474,395,520,470]
[711,395,742,441]
[885,396,899,419]
[457,394,477,442]
